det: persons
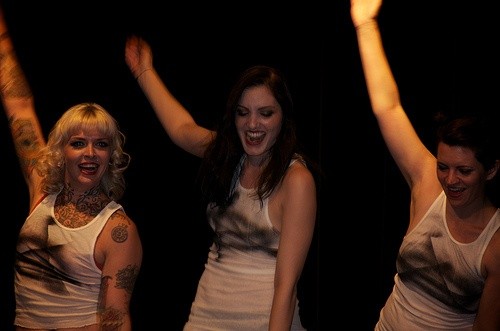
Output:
[0,19,143,331]
[350,0,500,331]
[127,34,315,330]
